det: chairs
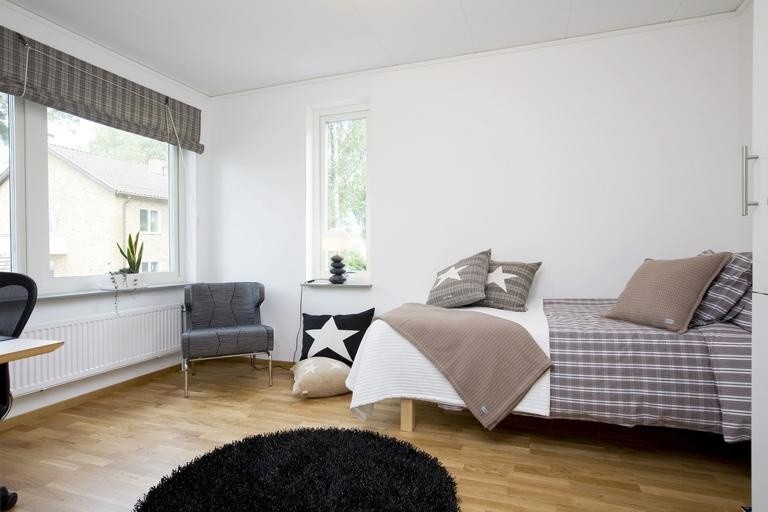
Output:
[0,272,37,422]
[179,280,274,399]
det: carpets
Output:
[133,427,461,512]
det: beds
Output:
[345,298,751,443]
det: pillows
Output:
[604,253,730,333]
[289,358,352,400]
[427,249,491,308]
[300,306,374,367]
[689,250,753,326]
[475,261,543,313]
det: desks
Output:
[0,336,64,365]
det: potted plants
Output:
[115,230,145,289]
